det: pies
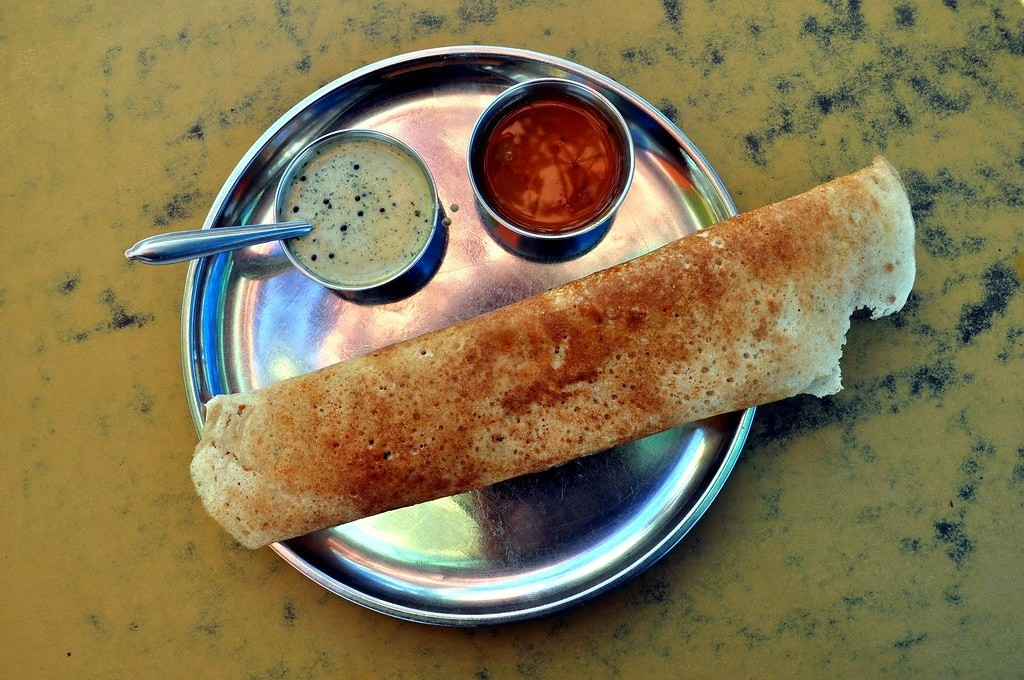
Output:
[188,155,917,548]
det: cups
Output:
[274,128,446,305]
[466,77,635,239]
